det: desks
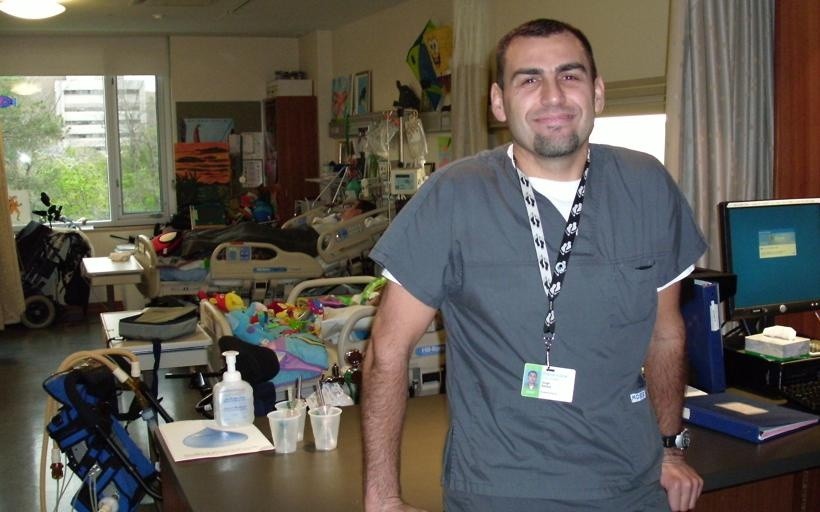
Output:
[100,307,213,466]
[150,387,819,511]
[82,254,144,311]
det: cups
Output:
[308,406,343,451]
[275,400,306,441]
[268,410,301,454]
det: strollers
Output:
[15,220,73,330]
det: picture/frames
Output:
[354,70,371,114]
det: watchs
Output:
[662,426,691,451]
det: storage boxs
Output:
[266,79,313,98]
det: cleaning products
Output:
[212,351,255,428]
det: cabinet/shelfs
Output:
[264,96,319,221]
[329,110,453,136]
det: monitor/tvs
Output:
[720,198,819,323]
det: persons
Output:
[525,371,537,389]
[359,19,708,511]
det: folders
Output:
[680,279,726,394]
[680,393,819,445]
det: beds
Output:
[164,275,447,418]
[135,201,397,308]
